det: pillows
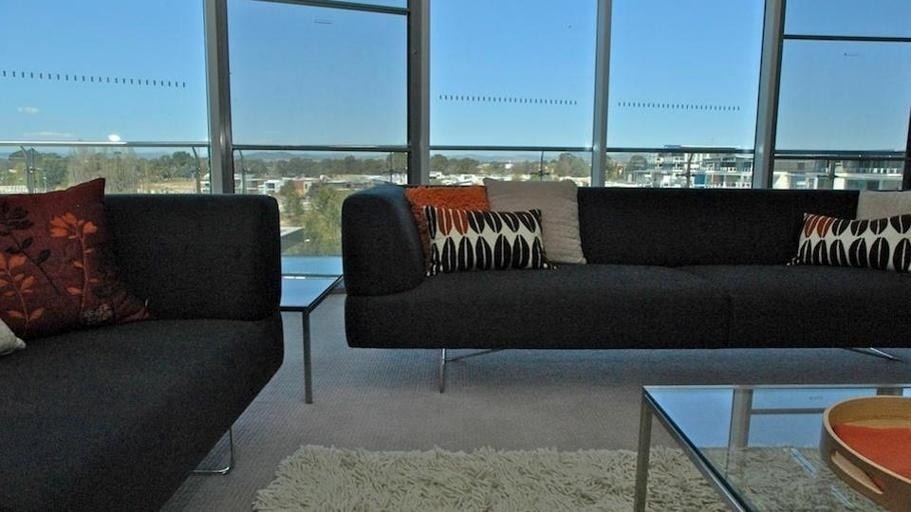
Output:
[406,180,589,272]
[786,188,910,268]
[2,177,150,340]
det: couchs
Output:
[0,192,285,510]
[338,183,911,389]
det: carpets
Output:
[244,444,883,511]
